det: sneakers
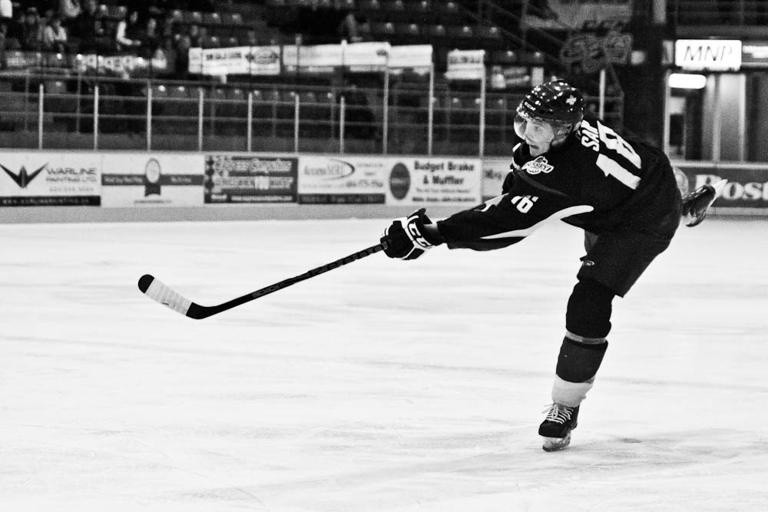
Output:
[538,402,579,439]
[681,185,716,227]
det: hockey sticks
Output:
[138,243,389,319]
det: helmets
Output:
[513,78,587,144]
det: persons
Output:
[381,78,718,440]
[40,1,208,78]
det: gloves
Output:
[380,207,435,261]
